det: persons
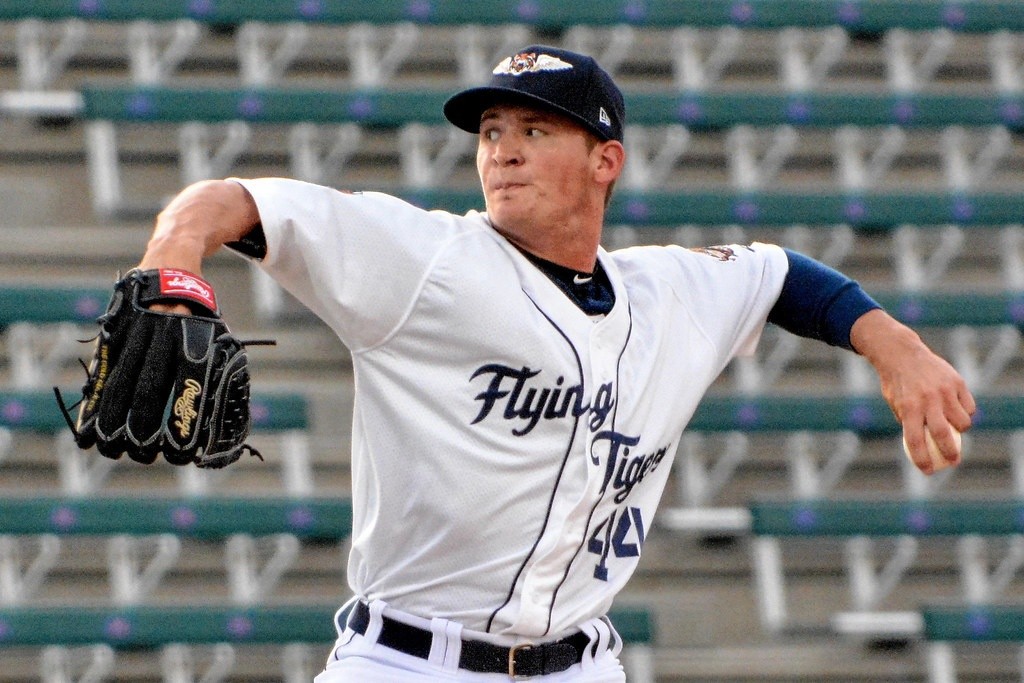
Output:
[53,46,977,683]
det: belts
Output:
[348,601,611,683]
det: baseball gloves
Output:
[51,264,279,471]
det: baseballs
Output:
[902,421,964,475]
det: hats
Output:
[443,45,625,143]
